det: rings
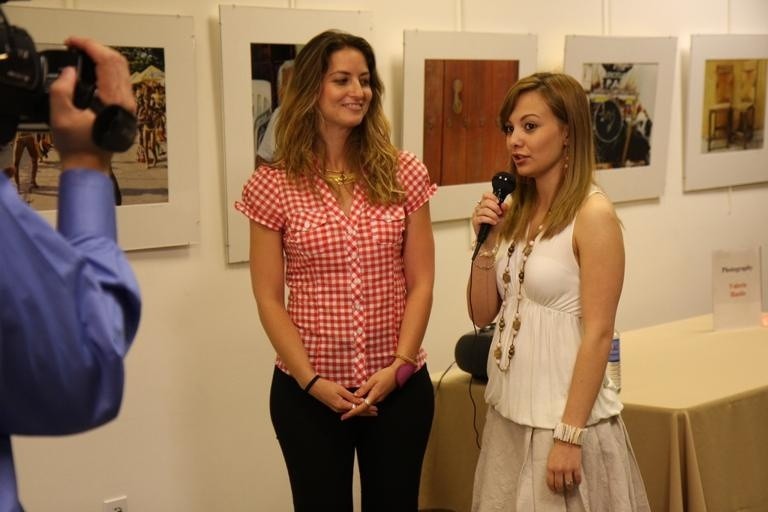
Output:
[564,480,572,484]
[351,403,358,411]
[363,399,373,407]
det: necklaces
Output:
[493,194,556,372]
[316,164,359,187]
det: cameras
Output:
[0,0,136,155]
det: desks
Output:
[432,315,768,512]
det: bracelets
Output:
[474,243,499,260]
[554,423,588,447]
[303,375,321,394]
[393,352,418,388]
[472,260,497,270]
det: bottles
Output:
[605,330,620,394]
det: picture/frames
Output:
[402,24,540,225]
[219,3,397,268]
[683,33,763,191]
[2,4,199,261]
[562,33,679,206]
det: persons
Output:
[1,8,142,512]
[13,131,38,195]
[135,81,164,170]
[234,30,434,512]
[36,129,53,163]
[467,72,651,512]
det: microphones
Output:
[470,171,516,257]
